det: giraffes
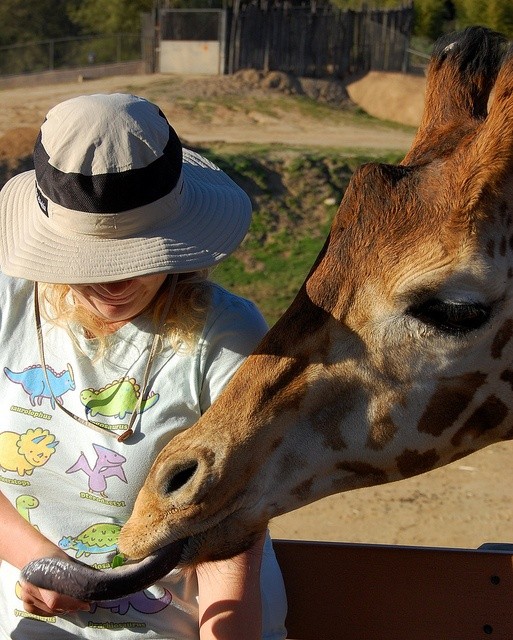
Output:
[21,27,513,604]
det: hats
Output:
[0,93,252,285]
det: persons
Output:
[0,92,287,640]
